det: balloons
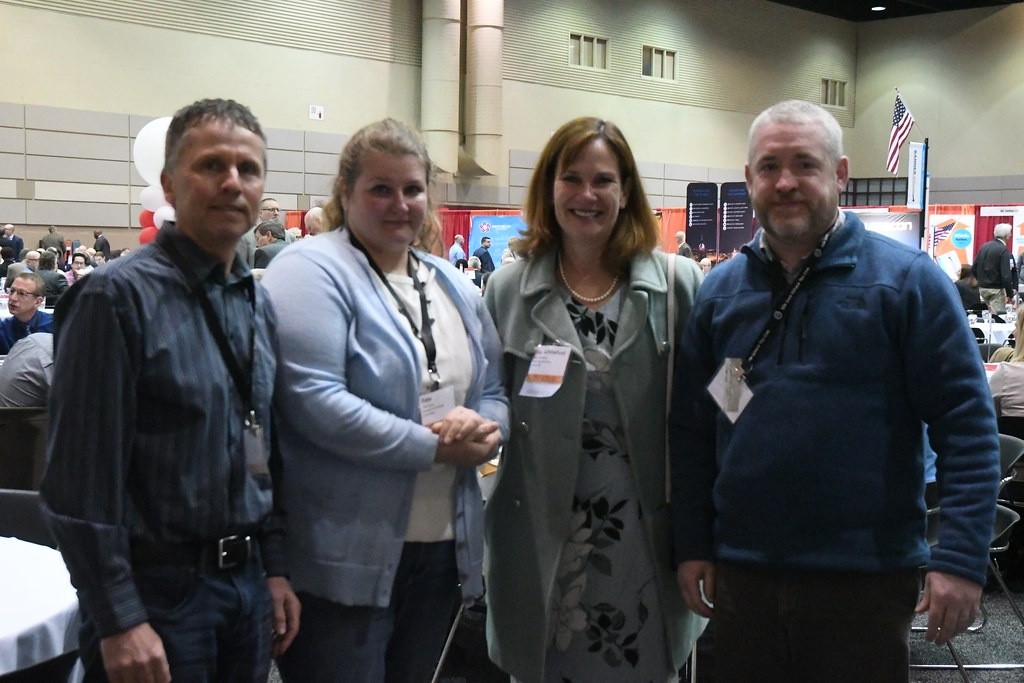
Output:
[139,210,155,228]
[132,117,173,185]
[140,186,165,211]
[139,226,158,243]
[153,205,176,229]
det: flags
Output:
[929,221,956,247]
[886,93,915,176]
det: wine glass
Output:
[981,310,990,326]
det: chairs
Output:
[0,406,51,490]
[915,414,1024,683]
[971,327,1019,363]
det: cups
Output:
[1006,315,1014,324]
[1005,304,1013,314]
[968,314,977,328]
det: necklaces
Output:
[559,250,618,301]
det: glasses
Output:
[261,207,282,213]
[6,287,41,298]
[29,258,40,262]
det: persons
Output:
[0,222,130,408]
[700,258,712,276]
[238,199,328,270]
[487,117,710,683]
[952,222,1024,363]
[36,99,302,683]
[502,236,518,264]
[669,101,1001,683]
[474,237,495,271]
[674,232,691,256]
[449,234,466,264]
[469,256,484,288]
[262,119,508,683]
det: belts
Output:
[128,532,260,574]
